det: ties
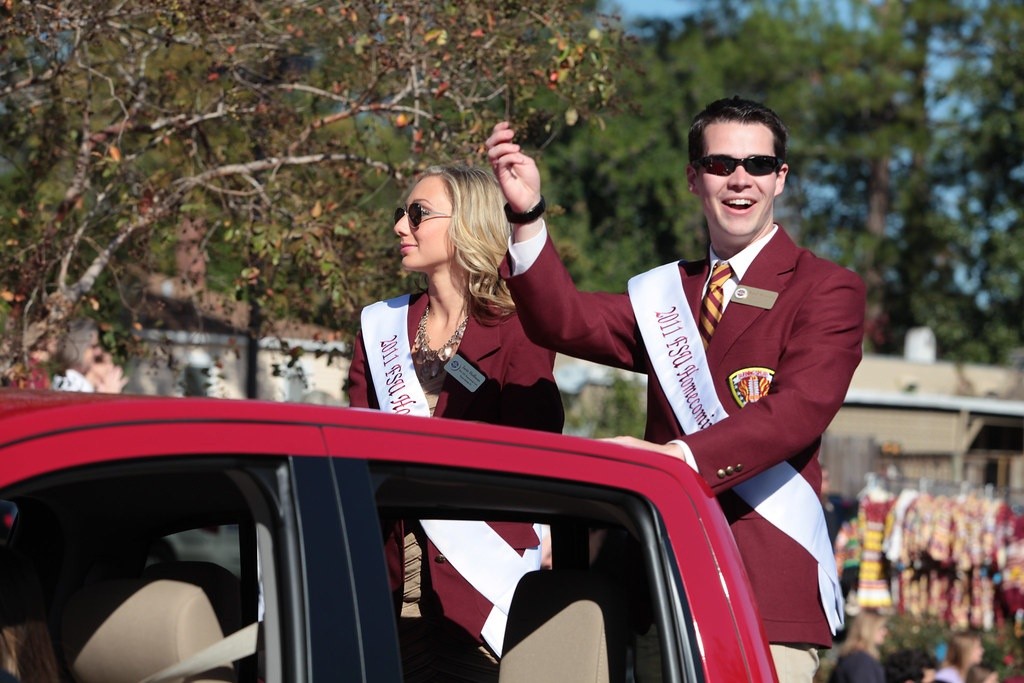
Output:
[699,263,736,354]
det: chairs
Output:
[143,562,241,669]
[498,572,628,683]
[61,560,242,683]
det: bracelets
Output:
[504,196,545,224]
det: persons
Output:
[485,96,866,683]
[820,467,847,553]
[885,650,935,683]
[828,609,887,683]
[933,633,1001,683]
[346,163,565,652]
[10,312,129,395]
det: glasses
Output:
[394,203,451,228]
[692,155,784,177]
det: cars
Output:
[0,387,780,681]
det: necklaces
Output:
[415,306,468,381]
